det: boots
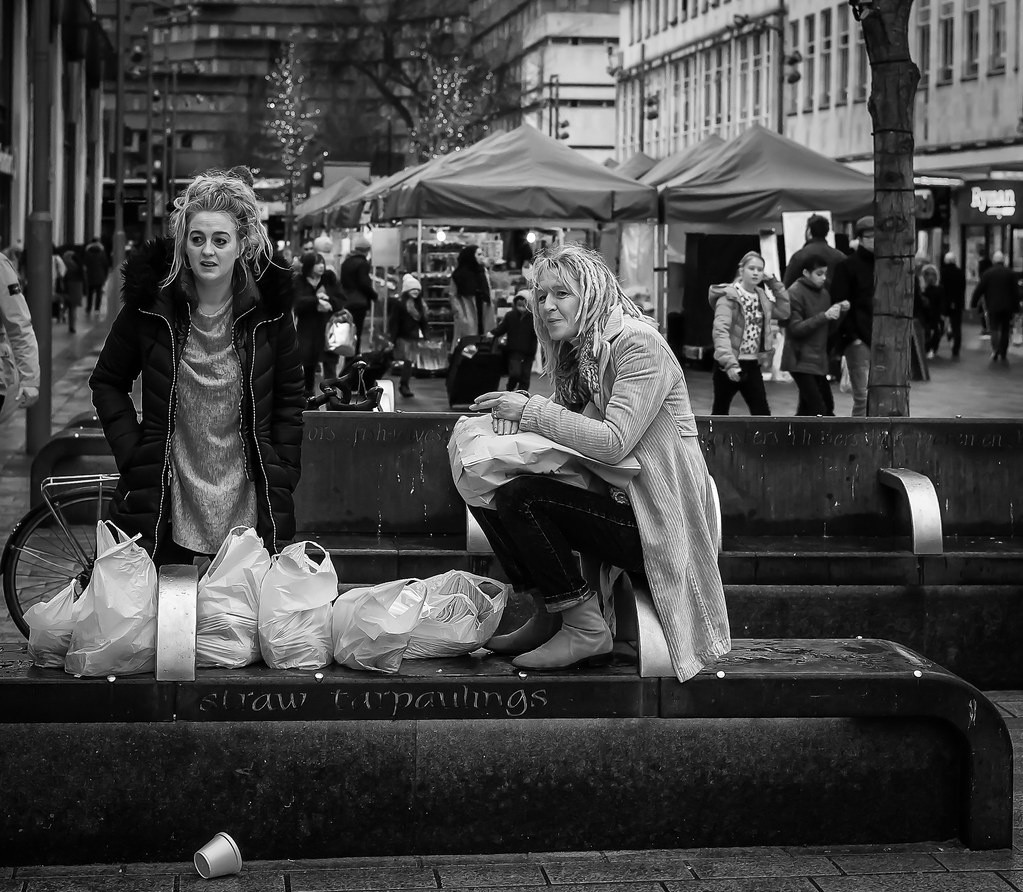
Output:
[481,591,560,652]
[512,587,613,673]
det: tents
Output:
[295,120,874,341]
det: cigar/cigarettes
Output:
[475,400,480,404]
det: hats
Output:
[854,215,874,234]
[400,274,422,293]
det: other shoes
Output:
[993,353,1007,362]
[399,383,415,397]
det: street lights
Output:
[126,8,206,245]
[606,44,659,152]
[726,0,802,134]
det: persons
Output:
[918,251,965,358]
[292,235,337,273]
[0,251,40,411]
[52,237,109,333]
[468,247,732,682]
[448,246,496,354]
[830,216,874,417]
[708,251,791,416]
[388,274,430,398]
[778,254,850,416]
[784,213,848,291]
[294,253,348,400]
[486,295,538,399]
[88,167,305,571]
[970,247,1020,363]
[340,236,378,351]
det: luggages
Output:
[445,329,505,406]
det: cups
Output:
[193,832,243,879]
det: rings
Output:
[494,411,497,418]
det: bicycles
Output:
[0,354,384,639]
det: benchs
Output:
[0,645,1015,865]
[292,411,1023,688]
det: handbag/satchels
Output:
[447,409,643,510]
[23,517,512,674]
[324,312,357,355]
